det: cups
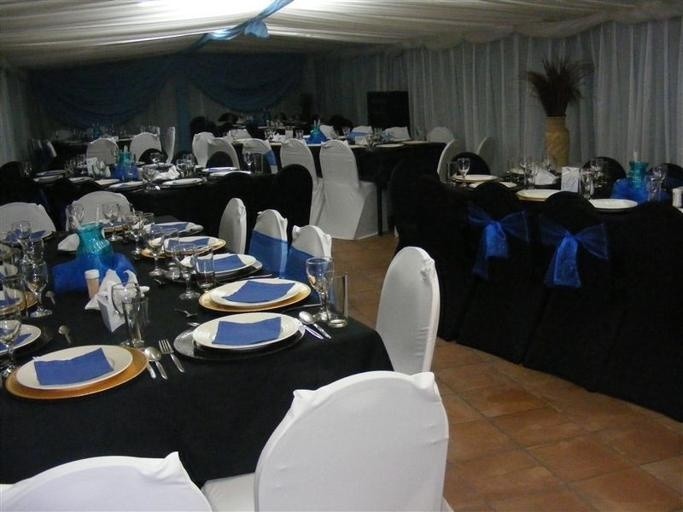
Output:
[323,271,349,328]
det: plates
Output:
[16,344,134,390]
[5,348,150,401]
[209,259,263,282]
[196,253,256,277]
[31,161,252,189]
[451,167,640,214]
[198,281,312,312]
[210,278,300,308]
[192,312,300,353]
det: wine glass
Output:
[75,154,196,186]
[0,202,216,378]
[305,257,336,322]
[457,155,667,201]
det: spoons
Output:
[299,311,332,340]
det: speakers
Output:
[366,90,410,127]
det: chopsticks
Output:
[172,318,305,363]
